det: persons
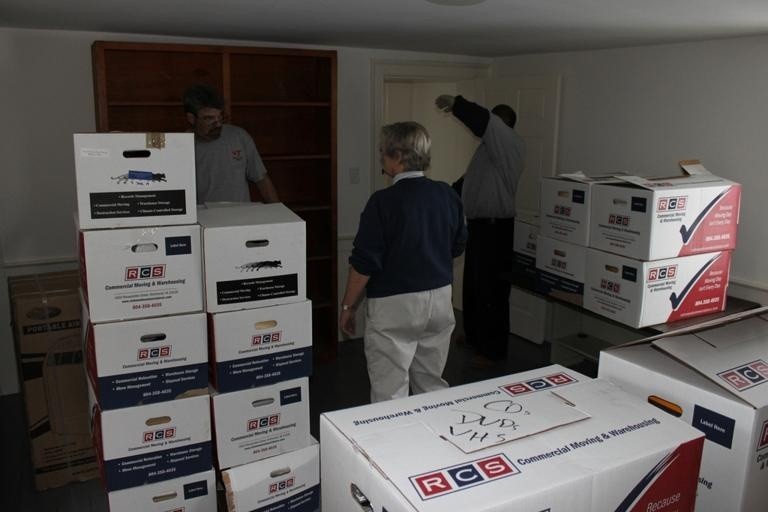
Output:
[339,120,468,404]
[435,94,526,369]
[183,85,281,205]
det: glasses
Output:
[192,111,230,132]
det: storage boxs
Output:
[538,171,658,248]
[511,210,546,276]
[211,375,311,472]
[320,364,705,511]
[78,287,210,408]
[196,201,307,313]
[209,299,311,392]
[535,234,597,296]
[74,210,205,322]
[85,372,214,492]
[587,157,743,260]
[105,469,217,510]
[598,307,767,512]
[217,434,319,512]
[74,131,198,230]
[11,269,104,492]
[584,247,732,328]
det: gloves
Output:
[434,93,455,113]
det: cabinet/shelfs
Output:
[92,41,338,362]
[509,278,768,366]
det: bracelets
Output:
[341,303,356,312]
